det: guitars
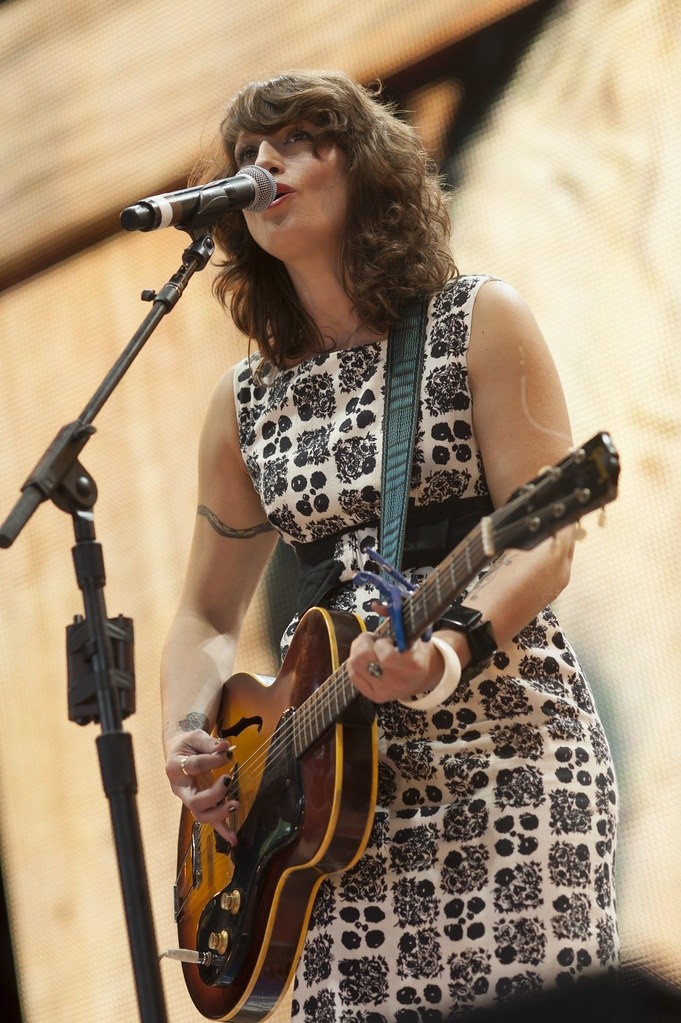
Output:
[173,430,621,1023]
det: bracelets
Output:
[397,637,461,711]
[437,604,498,684]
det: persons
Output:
[160,69,619,1023]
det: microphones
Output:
[120,164,278,232]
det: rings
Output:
[368,662,382,677]
[180,755,188,775]
